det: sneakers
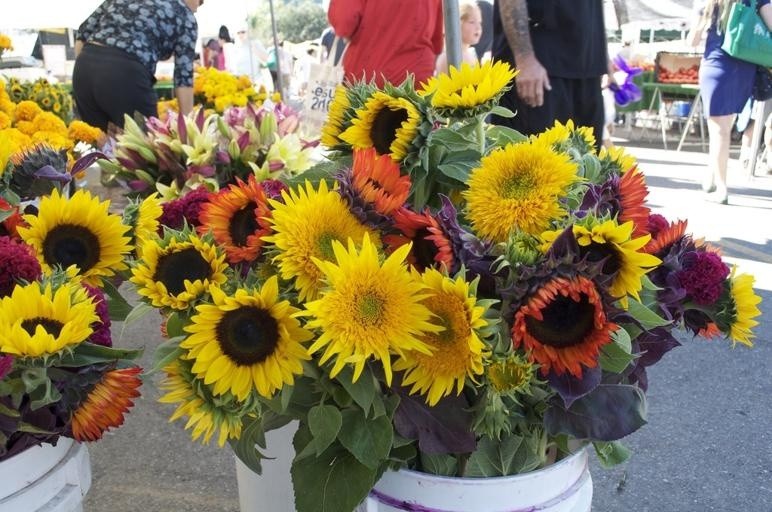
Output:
[700,176,729,204]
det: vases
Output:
[233,416,359,507]
[3,434,93,508]
[365,451,594,510]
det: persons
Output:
[698,0,772,204]
[328,0,444,93]
[204,25,348,116]
[490,1,606,159]
[433,0,494,75]
[73,0,203,130]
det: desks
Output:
[639,81,706,152]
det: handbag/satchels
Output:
[304,35,344,121]
[721,0,772,68]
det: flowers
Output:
[76,101,320,195]
[157,65,283,119]
[129,59,762,509]
[0,93,101,173]
[3,189,143,455]
[3,72,74,125]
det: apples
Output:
[660,68,697,80]
